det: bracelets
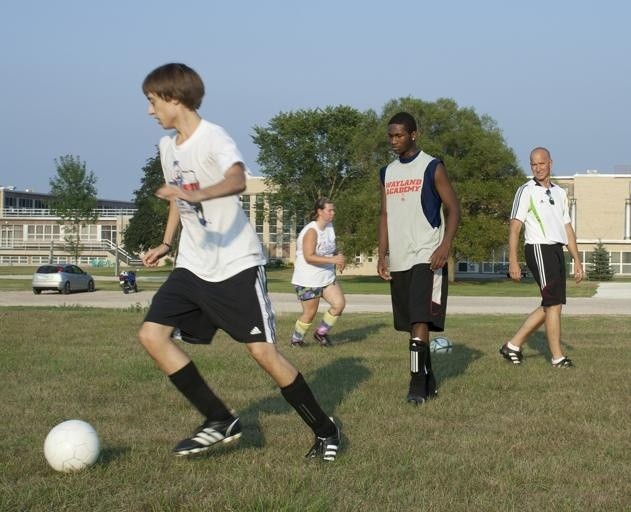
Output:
[159,240,172,251]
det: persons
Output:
[136,61,343,467]
[496,146,584,371]
[373,111,462,410]
[290,196,346,350]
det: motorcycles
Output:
[118,270,139,296]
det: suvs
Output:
[32,263,96,297]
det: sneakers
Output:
[290,336,304,347]
[313,329,331,346]
[172,415,243,457]
[500,343,523,364]
[407,376,437,403]
[316,416,341,461]
[553,358,573,369]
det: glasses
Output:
[545,189,555,205]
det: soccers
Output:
[429,337,452,354]
[44,419,100,473]
